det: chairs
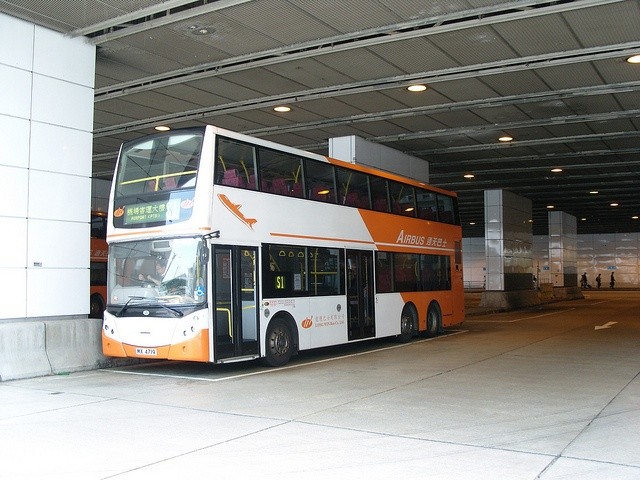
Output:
[269,177,289,196]
[372,198,387,212]
[292,183,304,199]
[341,192,359,208]
[391,201,401,214]
[162,177,177,190]
[222,168,242,188]
[248,175,266,192]
[310,185,329,202]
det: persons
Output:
[579,273,587,289]
[610,272,615,288]
[597,274,601,288]
[138,261,166,286]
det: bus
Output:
[88,178,112,318]
[102,124,465,368]
